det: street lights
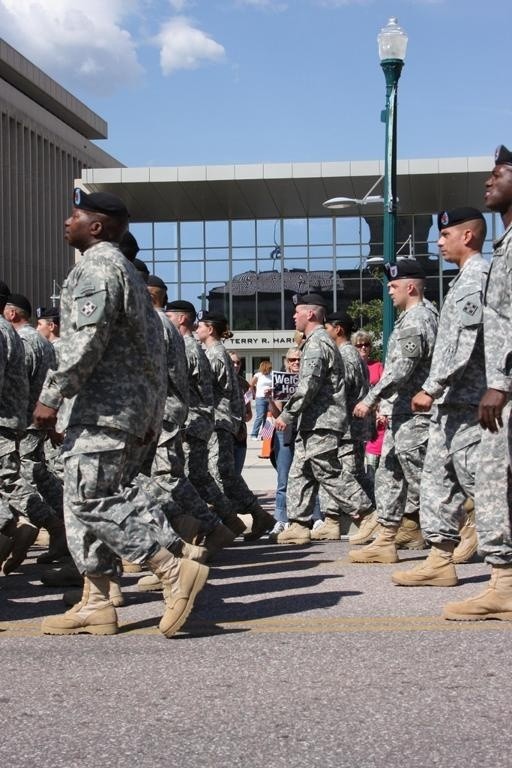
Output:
[322,16,409,367]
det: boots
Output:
[41,577,118,635]
[350,509,384,544]
[42,557,84,585]
[311,516,341,539]
[169,512,201,546]
[122,558,142,572]
[4,513,39,574]
[64,576,124,607]
[199,523,236,561]
[0,532,14,570]
[392,543,459,586]
[224,512,247,538]
[350,526,399,563]
[39,524,71,564]
[394,513,426,550]
[443,562,512,620]
[245,507,276,541]
[272,523,311,545]
[452,510,479,564]
[146,546,210,638]
[138,539,208,590]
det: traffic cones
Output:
[258,411,275,458]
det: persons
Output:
[276,295,378,546]
[0,283,41,581]
[137,274,235,552]
[30,187,207,640]
[250,360,276,438]
[306,314,376,539]
[2,292,56,556]
[159,301,247,541]
[186,310,276,544]
[348,330,388,502]
[348,260,438,566]
[447,146,512,623]
[31,306,63,364]
[268,347,303,539]
[395,206,490,586]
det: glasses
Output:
[289,357,302,362]
[355,343,371,347]
[233,360,241,365]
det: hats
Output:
[74,188,129,219]
[439,207,483,230]
[7,294,31,314]
[326,311,354,324]
[494,144,512,165]
[197,310,227,322]
[1,279,12,300]
[293,294,327,306]
[120,232,139,260]
[164,300,195,313]
[36,307,59,323]
[387,259,425,279]
[145,275,166,288]
[132,258,149,272]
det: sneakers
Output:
[249,435,258,441]
[268,522,293,543]
[310,520,324,531]
